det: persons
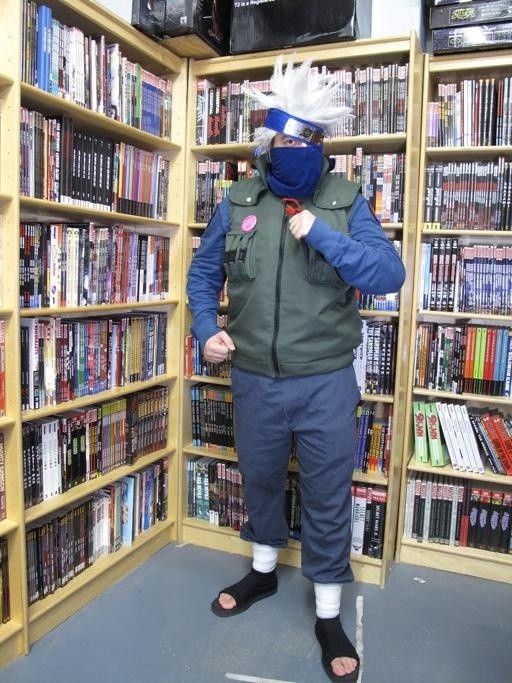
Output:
[181,107,410,683]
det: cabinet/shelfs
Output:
[177,31,423,590]
[393,48,512,585]
[0,0,188,670]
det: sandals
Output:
[314,616,359,683]
[210,568,277,617]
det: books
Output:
[21,1,168,220]
[191,382,235,452]
[356,240,402,311]
[352,484,387,558]
[194,60,408,144]
[329,147,406,224]
[185,315,231,378]
[355,402,393,477]
[284,476,301,540]
[352,318,397,395]
[194,148,259,222]
[402,76,511,554]
[221,278,229,302]
[0,215,169,624]
[188,455,248,531]
[191,237,201,257]
